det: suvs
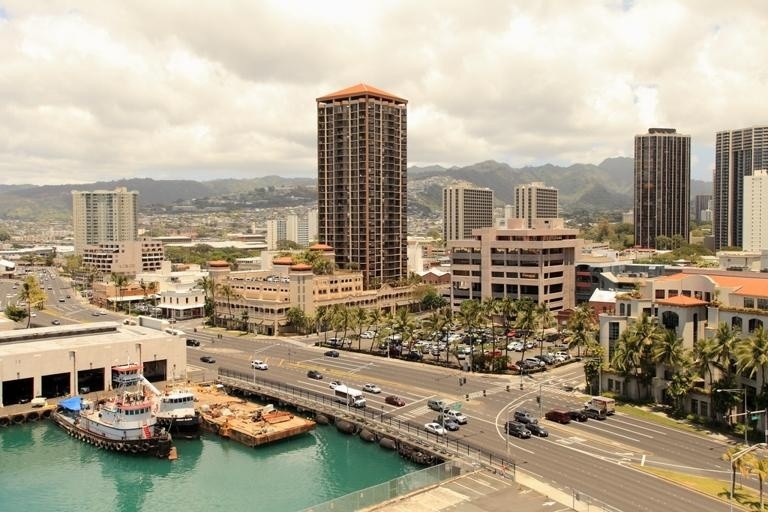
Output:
[447,409,467,424]
[428,398,450,412]
[363,382,381,394]
[251,359,268,370]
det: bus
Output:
[334,385,366,408]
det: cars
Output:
[78,384,90,394]
[424,421,447,436]
[324,314,573,376]
[240,273,293,284]
[10,268,107,326]
[384,395,405,406]
[5,397,28,404]
[200,356,216,363]
[329,380,345,389]
[308,370,323,379]
[433,415,459,431]
[186,338,200,346]
[167,317,177,323]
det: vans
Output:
[132,301,161,314]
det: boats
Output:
[51,363,172,458]
[31,395,47,407]
[155,388,204,440]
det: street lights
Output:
[730,442,767,511]
[717,388,748,447]
[571,356,602,396]
[505,399,533,461]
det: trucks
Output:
[504,394,615,439]
